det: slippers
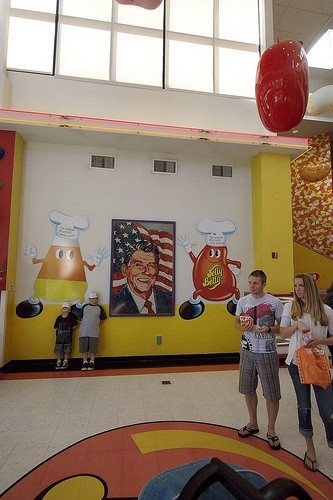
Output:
[304,451,317,472]
[238,426,259,438]
[266,432,281,450]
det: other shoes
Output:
[55,360,61,369]
[81,362,88,371]
[88,362,95,371]
[62,361,68,369]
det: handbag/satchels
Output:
[295,344,331,390]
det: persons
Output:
[234,269,286,450]
[75,292,107,371]
[54,302,78,369]
[279,272,333,470]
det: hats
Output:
[89,292,98,299]
[61,302,71,312]
